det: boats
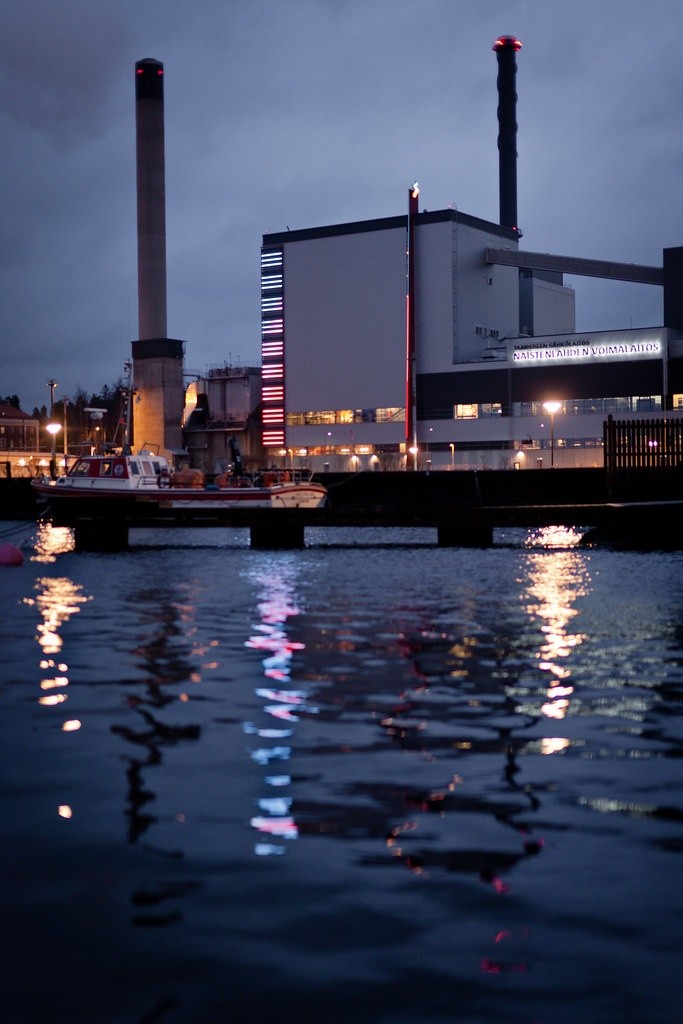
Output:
[31,359,328,508]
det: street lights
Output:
[450,444,455,471]
[426,428,433,471]
[353,454,358,472]
[46,380,61,466]
[543,400,559,468]
[288,449,292,469]
[62,398,70,455]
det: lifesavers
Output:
[157,472,173,490]
